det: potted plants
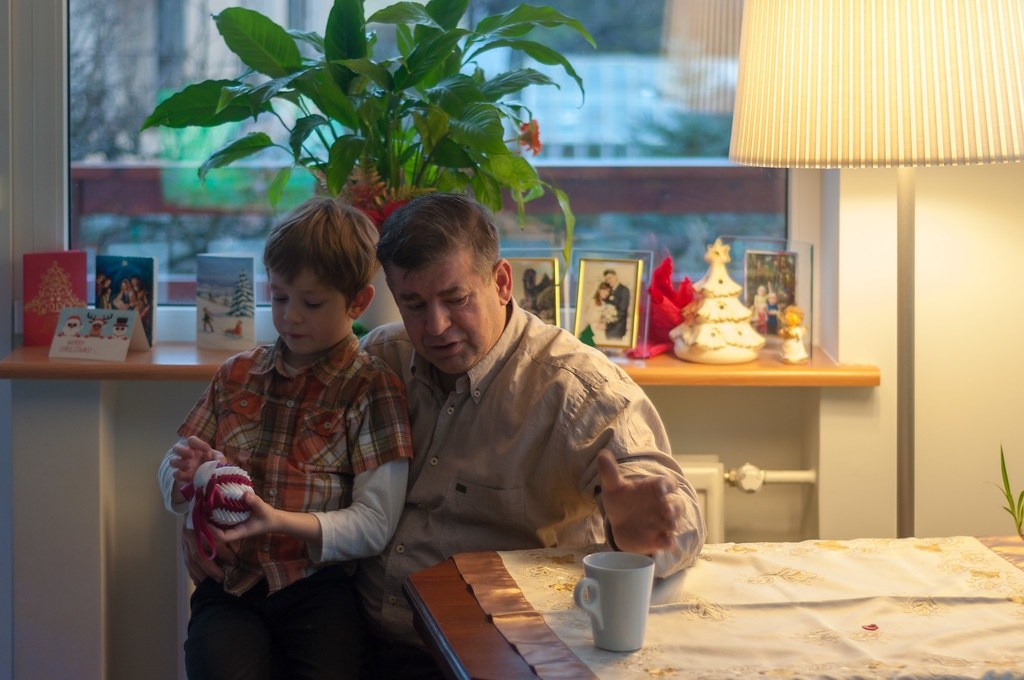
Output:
[139,1,595,334]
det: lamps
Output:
[729,1,1024,538]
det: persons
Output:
[602,270,630,340]
[358,190,707,680]
[159,199,412,679]
[586,282,611,341]
[778,305,811,365]
[753,279,779,332]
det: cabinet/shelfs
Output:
[0,334,881,387]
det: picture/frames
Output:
[722,236,816,359]
[568,249,652,359]
[501,248,570,333]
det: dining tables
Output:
[407,537,1024,680]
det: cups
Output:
[574,551,656,653]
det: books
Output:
[18,251,255,362]
